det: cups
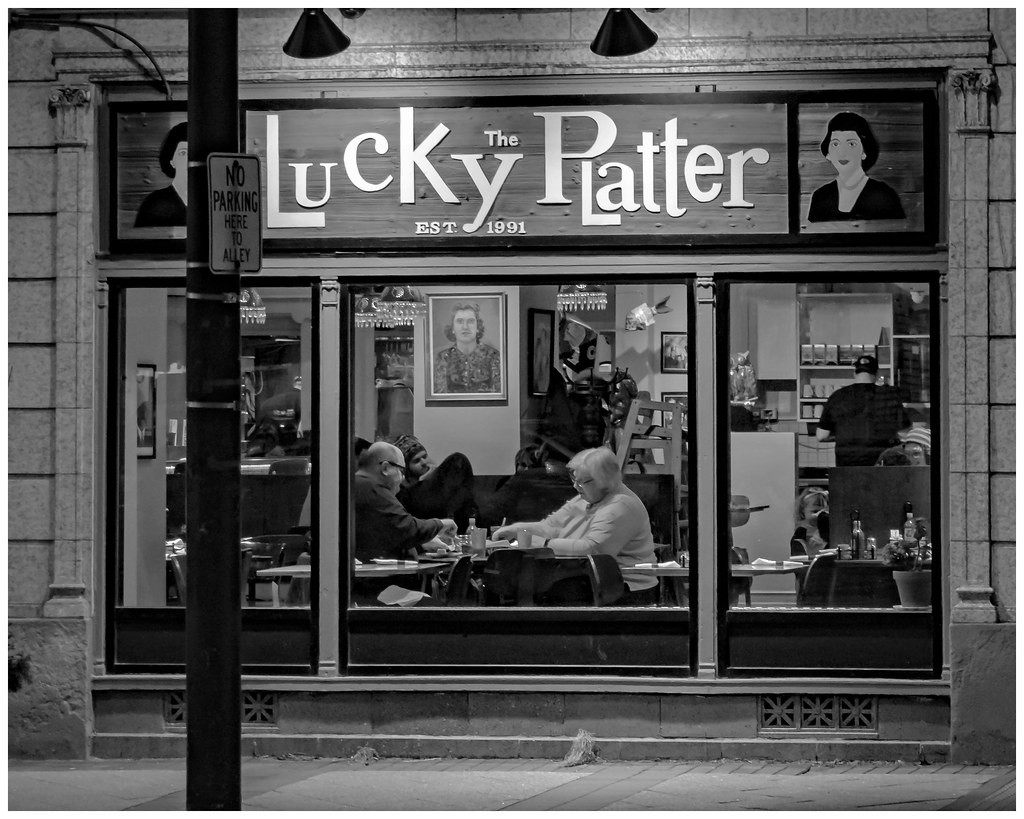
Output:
[490,526,504,540]
[801,385,847,419]
[517,531,532,548]
[470,526,487,558]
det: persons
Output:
[433,303,504,394]
[794,486,832,556]
[817,355,910,464]
[298,434,659,603]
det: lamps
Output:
[283,8,366,59]
[240,287,266,325]
[354,286,428,329]
[589,8,665,58]
[556,285,609,312]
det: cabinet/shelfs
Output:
[893,333,932,409]
[794,291,895,424]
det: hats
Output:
[393,434,425,466]
[856,356,878,373]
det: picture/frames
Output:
[526,306,555,398]
[660,331,688,374]
[423,291,509,402]
[135,363,157,460]
[660,391,689,443]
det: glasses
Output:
[380,460,407,475]
[570,474,594,489]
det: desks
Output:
[622,563,810,607]
[255,550,588,608]
[166,465,312,527]
[795,553,933,610]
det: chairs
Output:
[166,459,314,606]
[377,547,632,607]
[661,546,838,608]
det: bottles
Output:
[462,518,479,554]
[852,521,865,560]
[835,544,851,559]
[866,538,877,560]
[903,512,916,541]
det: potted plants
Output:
[887,518,933,609]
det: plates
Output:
[370,558,419,565]
